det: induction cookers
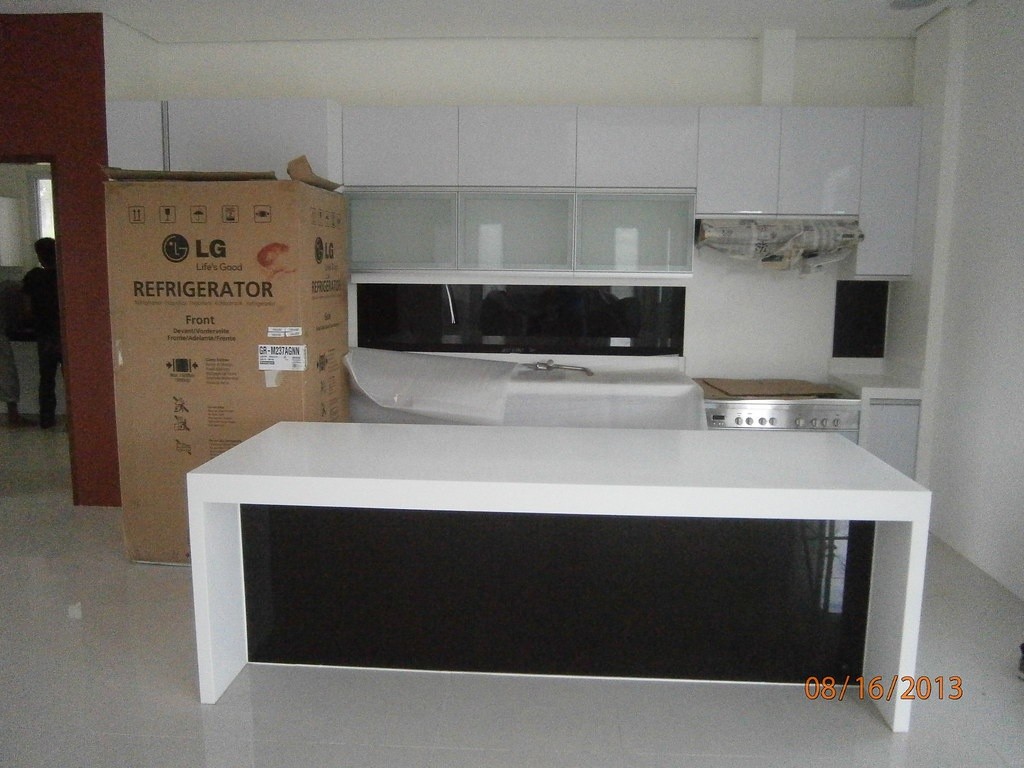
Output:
[693,378,861,430]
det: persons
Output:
[0,238,63,428]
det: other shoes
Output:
[8,414,37,429]
[40,416,61,429]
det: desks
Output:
[186,422,933,732]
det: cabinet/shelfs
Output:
[106,98,920,286]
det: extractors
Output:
[695,219,865,272]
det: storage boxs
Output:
[98,155,350,566]
